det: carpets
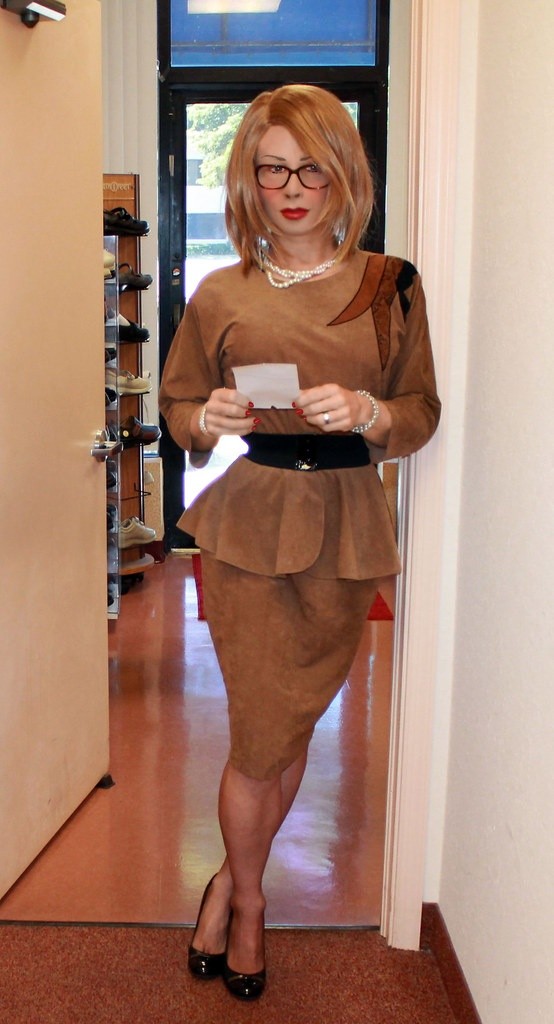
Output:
[191,553,396,621]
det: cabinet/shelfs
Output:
[104,235,122,619]
[103,173,153,594]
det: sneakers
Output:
[103,207,150,236]
[119,515,157,548]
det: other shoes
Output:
[108,415,162,445]
[105,368,152,394]
[117,261,153,289]
[105,312,150,344]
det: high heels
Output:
[187,872,231,980]
[224,907,267,1001]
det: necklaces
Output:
[262,237,342,288]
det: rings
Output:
[323,413,330,424]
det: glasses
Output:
[255,163,332,190]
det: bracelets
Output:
[350,390,380,433]
[198,403,216,438]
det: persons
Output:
[159,85,442,1002]
